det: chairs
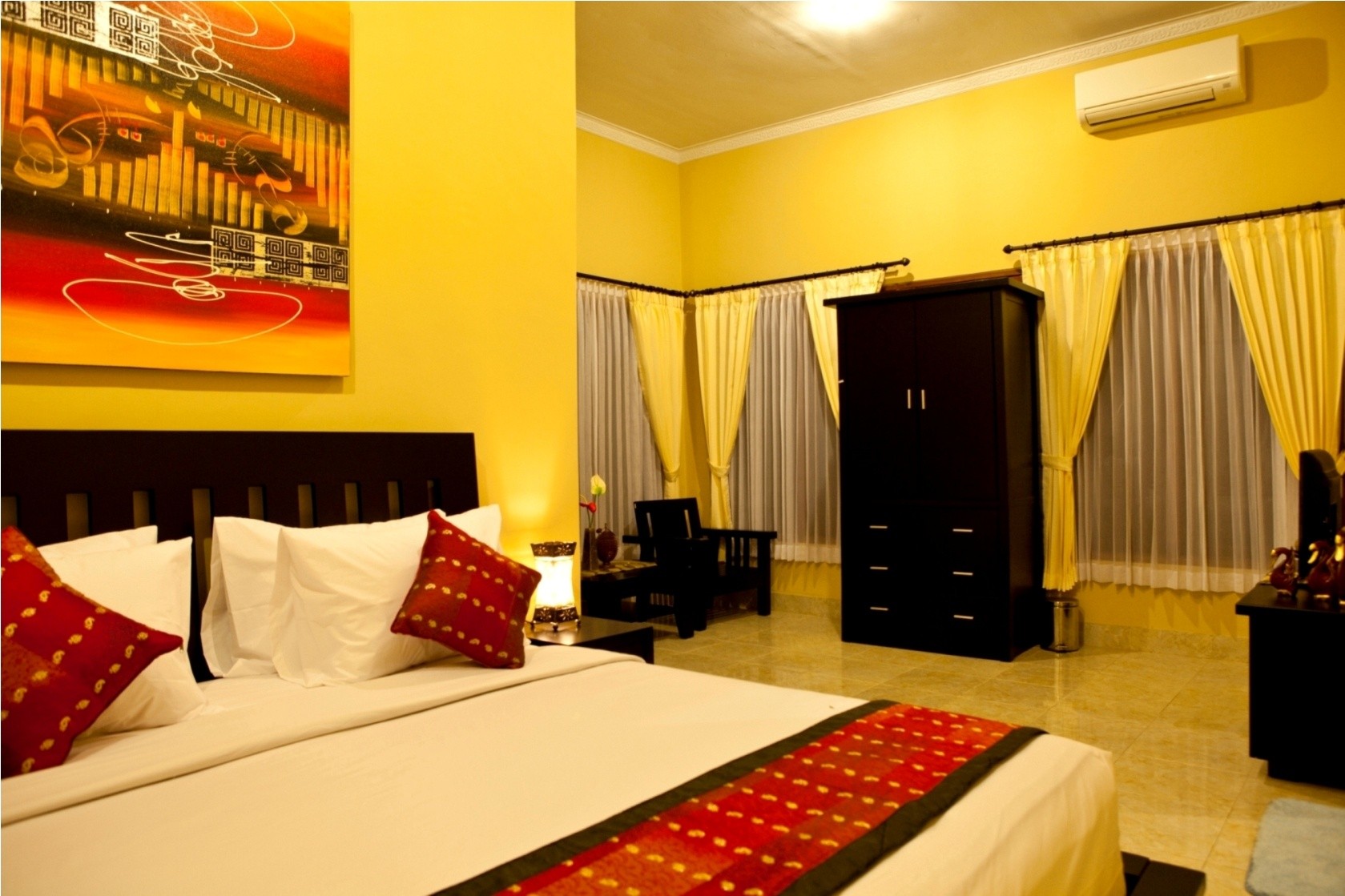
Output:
[635,499,779,632]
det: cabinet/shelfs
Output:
[822,274,1047,661]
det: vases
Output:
[582,527,598,570]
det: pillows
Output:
[1,509,551,777]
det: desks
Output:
[584,561,660,621]
[1235,574,1345,792]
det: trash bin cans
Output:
[1044,597,1078,650]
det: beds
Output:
[0,432,1210,896]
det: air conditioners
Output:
[1076,34,1247,134]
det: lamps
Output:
[530,541,583,632]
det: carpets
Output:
[1245,799,1345,896]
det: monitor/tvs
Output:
[1295,450,1344,580]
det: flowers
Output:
[580,475,606,528]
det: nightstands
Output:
[526,615,655,664]
